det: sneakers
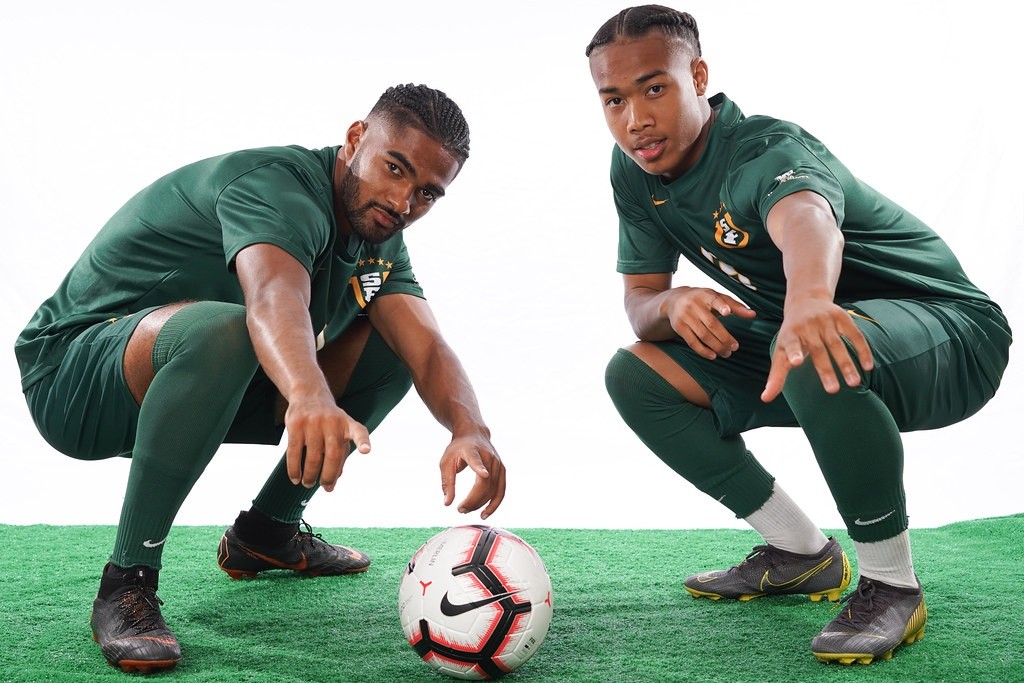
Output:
[216,521,370,581]
[82,578,184,672]
[809,578,925,663]
[682,536,851,607]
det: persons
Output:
[586,4,1013,664]
[13,82,507,673]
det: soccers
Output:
[398,524,554,681]
